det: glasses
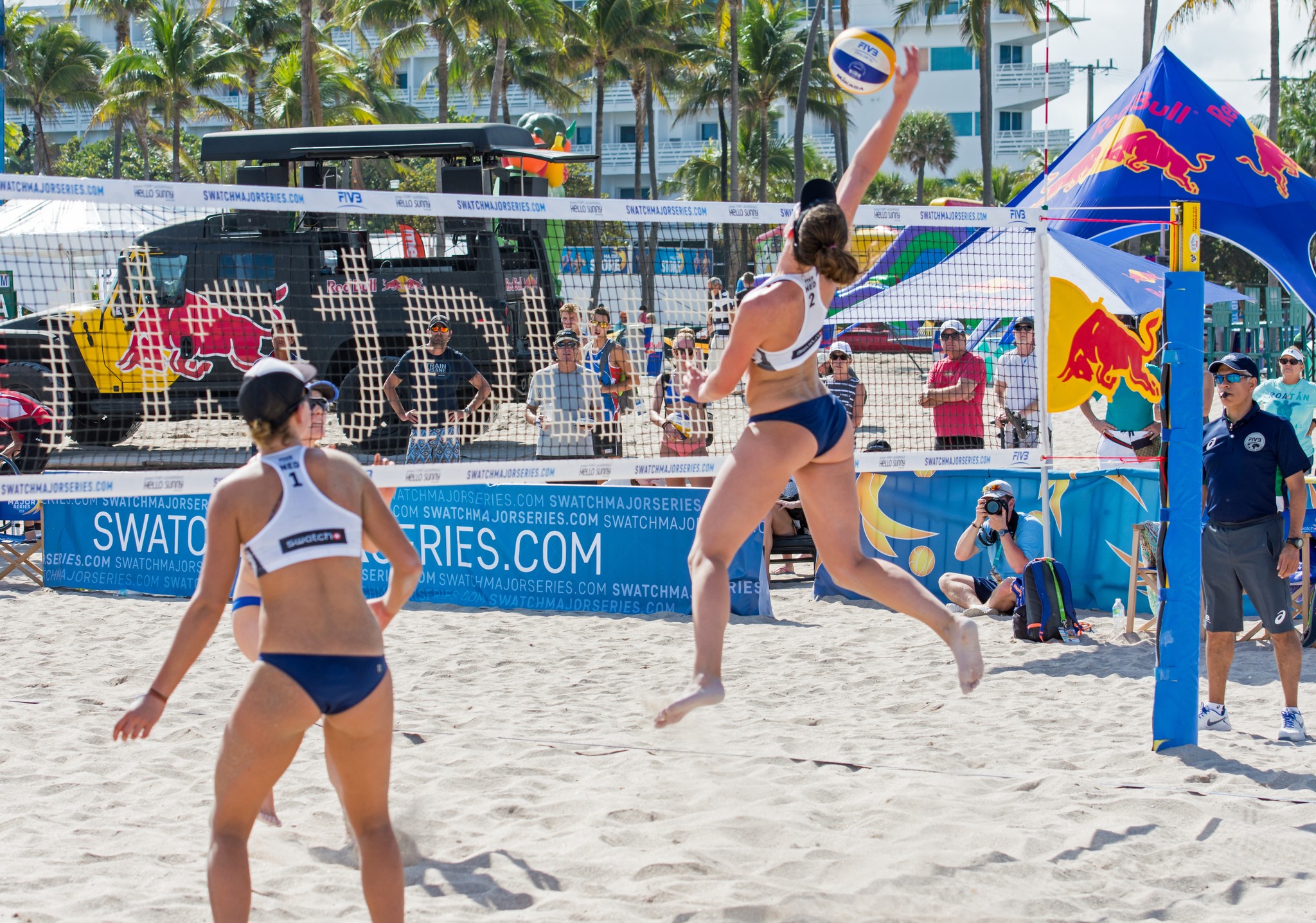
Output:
[1015,325,1032,331]
[830,354,851,360]
[1213,373,1254,384]
[792,202,800,227]
[646,317,650,319]
[981,485,1013,495]
[431,326,449,333]
[591,320,608,328]
[308,396,328,411]
[673,348,693,356]
[286,387,309,414]
[557,342,579,348]
[1279,358,1303,365]
[941,332,965,341]
[709,288,713,291]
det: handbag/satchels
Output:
[1131,433,1161,463]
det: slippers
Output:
[793,554,803,559]
[771,567,795,575]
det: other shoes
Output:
[946,603,967,613]
[962,605,999,617]
[13,537,42,546]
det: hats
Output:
[816,352,829,368]
[620,312,627,318]
[270,323,294,336]
[291,364,318,384]
[793,178,840,256]
[1208,353,1259,380]
[829,341,852,356]
[428,315,451,329]
[940,320,964,334]
[1280,348,1304,364]
[1013,317,1034,328]
[555,328,578,344]
[304,380,339,403]
[862,439,892,452]
[236,356,305,421]
[979,479,1013,499]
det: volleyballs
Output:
[828,26,896,94]
[666,412,692,442]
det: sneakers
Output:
[1198,702,1232,731]
[1278,707,1306,742]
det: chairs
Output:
[762,533,818,584]
[1125,521,1161,634]
[1239,474,1316,642]
[0,497,45,588]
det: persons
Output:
[649,328,715,489]
[915,317,1058,621]
[655,39,987,733]
[562,249,586,274]
[108,317,424,923]
[525,300,665,490]
[692,251,712,275]
[209,371,402,805]
[630,477,666,487]
[749,338,891,580]
[0,391,61,543]
[1069,314,1316,744]
[697,270,759,351]
[383,314,491,470]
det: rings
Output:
[1150,431,1153,434]
[1102,429,1106,434]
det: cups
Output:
[539,403,553,421]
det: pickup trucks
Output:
[1,121,598,459]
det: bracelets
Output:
[1091,417,1098,426]
[972,520,982,529]
[1313,419,1316,427]
[146,688,170,705]
[662,421,671,429]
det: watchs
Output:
[999,529,1009,536]
[464,407,472,416]
[1284,537,1304,549]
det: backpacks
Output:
[1010,557,1085,644]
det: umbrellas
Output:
[819,218,1262,558]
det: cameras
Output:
[983,497,1007,516]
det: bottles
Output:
[1112,598,1125,634]
